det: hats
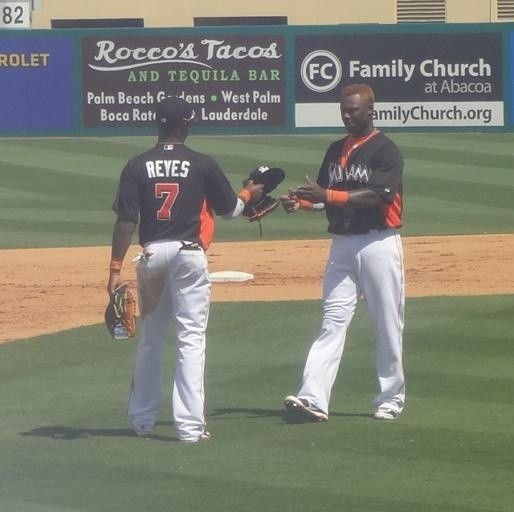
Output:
[156,96,196,126]
[249,165,285,194]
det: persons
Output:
[278,82,405,421]
[107,96,265,442]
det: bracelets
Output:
[109,258,122,273]
[299,197,314,212]
[326,188,349,208]
[238,188,251,205]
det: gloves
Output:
[132,247,154,262]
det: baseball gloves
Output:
[241,166,285,235]
[105,283,136,340]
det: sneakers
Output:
[282,394,329,422]
[129,419,154,436]
[374,403,399,421]
[180,432,210,443]
